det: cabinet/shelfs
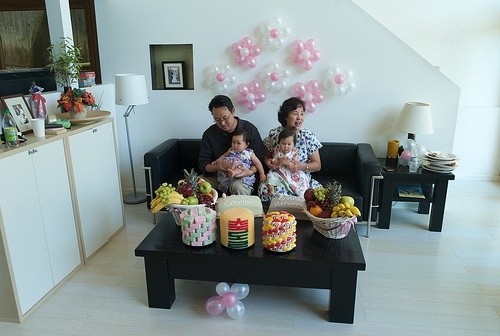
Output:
[0,117,127,322]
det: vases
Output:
[70,108,87,118]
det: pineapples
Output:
[324,180,341,210]
[182,168,201,195]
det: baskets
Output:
[170,188,218,226]
[303,201,357,239]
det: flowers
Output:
[56,88,99,115]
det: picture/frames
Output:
[162,61,186,89]
[0,93,35,135]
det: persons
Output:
[15,106,28,125]
[198,95,265,195]
[267,129,310,196]
[211,128,266,191]
[258,97,324,201]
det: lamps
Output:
[115,73,147,204]
[395,102,433,142]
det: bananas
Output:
[331,202,361,218]
[150,191,183,213]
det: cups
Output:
[30,119,45,138]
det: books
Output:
[397,186,426,199]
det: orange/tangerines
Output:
[309,205,322,215]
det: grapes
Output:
[307,187,331,210]
[196,192,214,208]
[155,182,175,202]
[181,198,190,205]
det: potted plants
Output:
[44,37,84,114]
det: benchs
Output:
[144,138,384,238]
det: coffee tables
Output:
[135,212,366,324]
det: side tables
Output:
[378,158,455,232]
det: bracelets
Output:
[305,164,308,171]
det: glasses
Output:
[214,112,232,123]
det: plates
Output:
[422,152,459,174]
[56,110,111,124]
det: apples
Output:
[340,196,355,205]
[179,182,212,205]
[304,189,316,201]
[320,211,331,218]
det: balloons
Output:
[208,16,354,113]
[206,282,249,320]
[399,139,425,169]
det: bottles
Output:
[30,88,48,120]
[2,110,19,149]
[409,147,418,172]
